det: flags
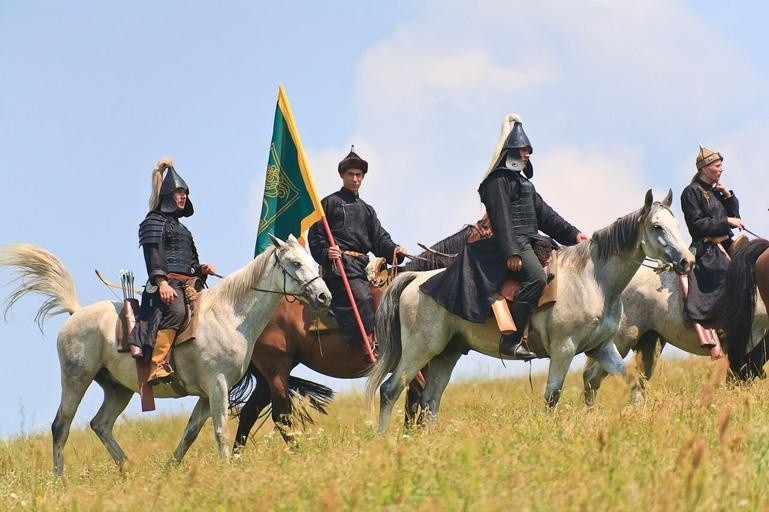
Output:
[252,83,325,260]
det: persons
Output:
[476,147,594,361]
[679,143,745,340]
[137,188,215,385]
[307,145,407,363]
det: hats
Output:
[695,142,722,167]
[338,146,368,176]
[148,157,194,217]
[487,112,533,178]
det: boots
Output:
[500,301,536,358]
[147,329,175,384]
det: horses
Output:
[229,214,494,454]
[358,188,696,435]
[0,231,333,479]
[723,239,769,390]
[583,264,769,407]
